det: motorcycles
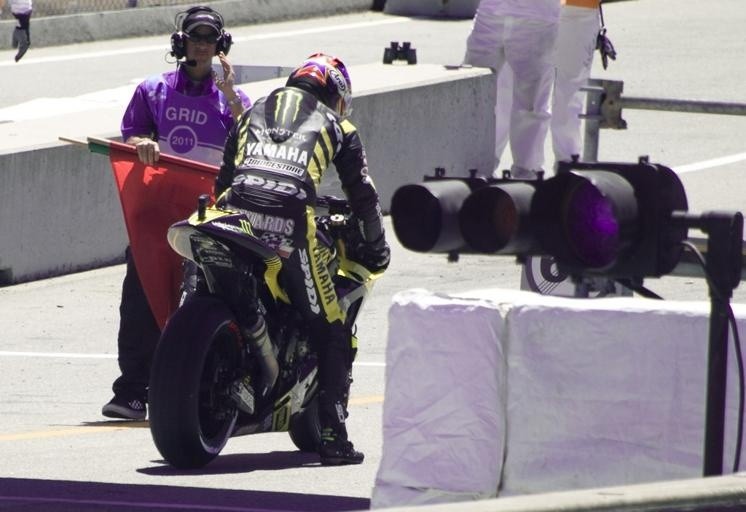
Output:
[147,194,390,468]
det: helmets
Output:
[286,52,352,116]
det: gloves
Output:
[596,32,617,69]
[368,242,391,267]
[11,11,31,63]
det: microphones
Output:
[175,59,199,67]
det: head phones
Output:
[170,6,233,59]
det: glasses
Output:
[189,32,217,44]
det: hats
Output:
[183,5,223,36]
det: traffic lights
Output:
[391,155,688,277]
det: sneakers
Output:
[321,428,364,466]
[102,395,147,419]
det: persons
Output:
[464,1,600,179]
[102,6,251,419]
[215,53,386,465]
[11,0,33,61]
